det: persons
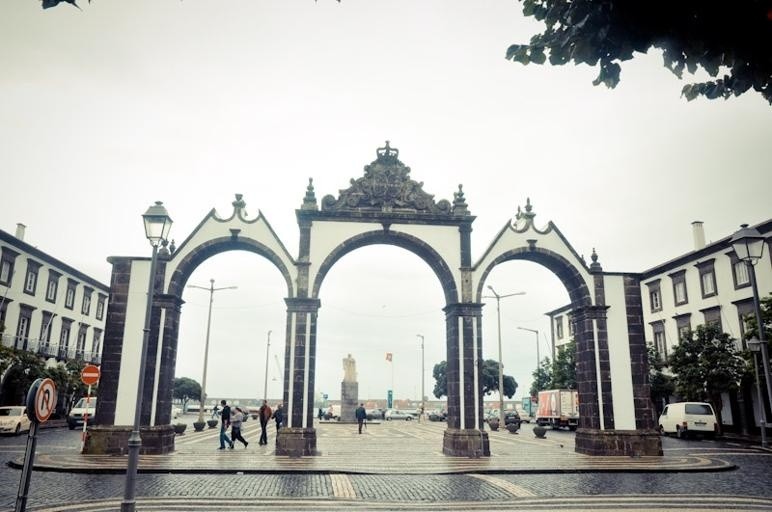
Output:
[217,399,234,450]
[258,400,272,445]
[355,403,366,434]
[319,405,322,420]
[211,403,219,419]
[229,408,248,449]
[342,353,357,382]
[271,404,283,443]
[548,392,558,416]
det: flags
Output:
[385,352,392,363]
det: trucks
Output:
[535,388,579,432]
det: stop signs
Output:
[80,365,100,385]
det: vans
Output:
[67,395,98,432]
[654,400,719,438]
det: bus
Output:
[186,405,223,415]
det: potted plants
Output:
[505,422,520,434]
[488,419,500,432]
[532,426,548,439]
[171,408,260,435]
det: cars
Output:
[365,397,538,433]
[0,405,33,437]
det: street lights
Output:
[748,336,769,449]
[117,192,177,511]
[264,328,273,403]
[414,333,425,415]
[729,219,772,413]
[179,278,240,423]
[480,283,528,432]
[515,325,541,391]
